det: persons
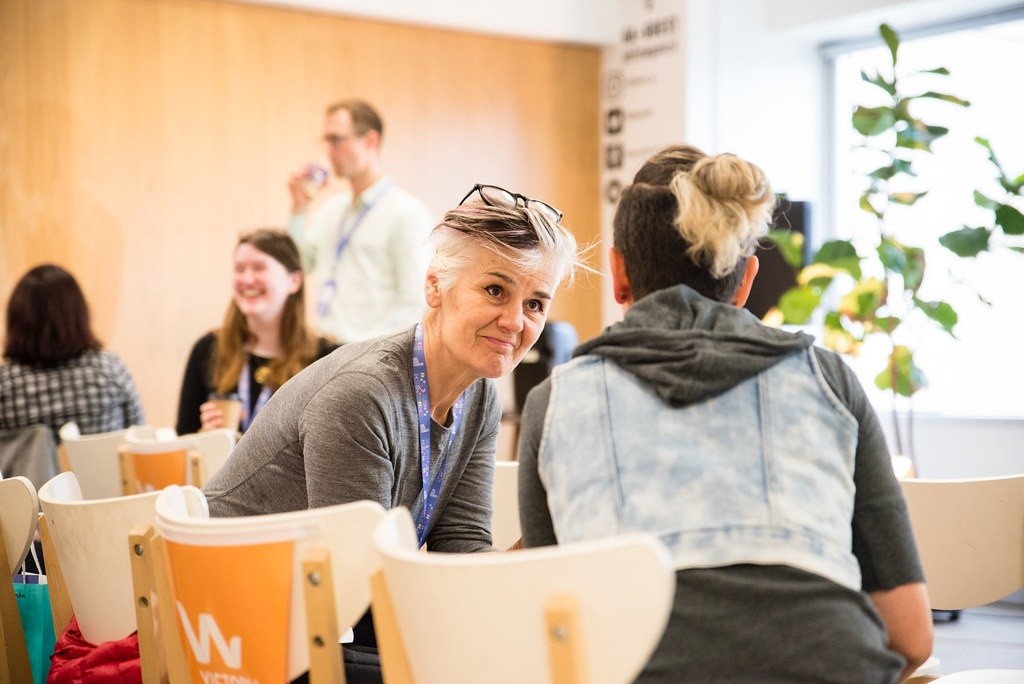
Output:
[287,98,436,349]
[194,196,578,684]
[176,228,342,442]
[2,264,145,446]
[517,142,935,683]
[751,205,882,356]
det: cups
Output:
[207,392,241,436]
[301,165,328,198]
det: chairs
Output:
[0,427,1024,684]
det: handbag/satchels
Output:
[12,541,57,684]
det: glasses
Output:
[457,183,563,225]
